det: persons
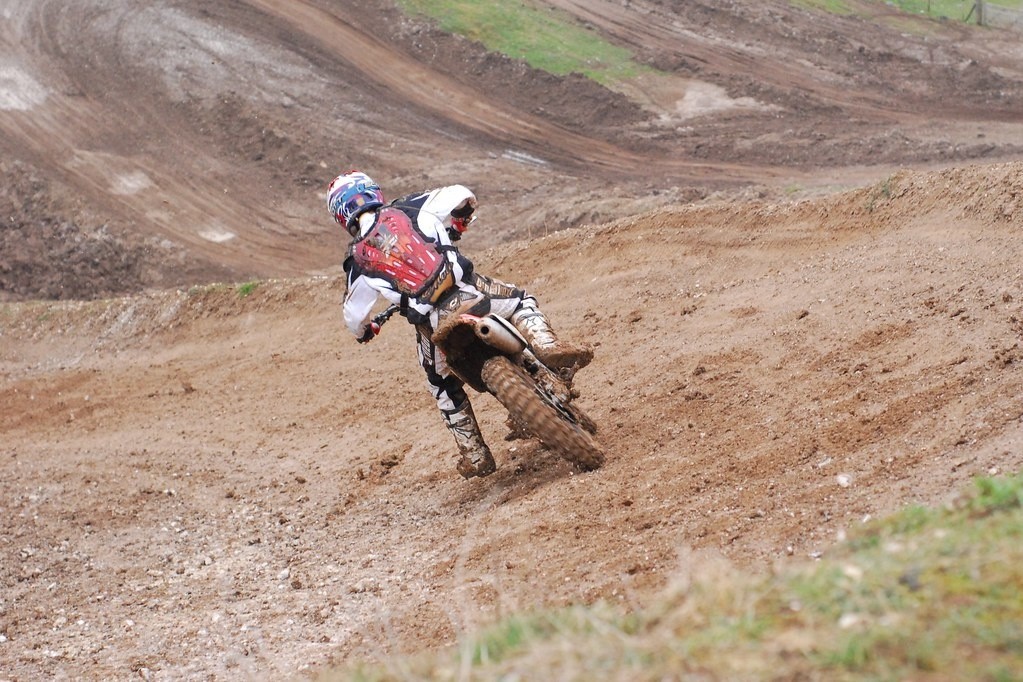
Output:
[326,169,594,479]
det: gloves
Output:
[451,216,472,232]
[356,324,375,344]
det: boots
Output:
[440,393,496,480]
[511,306,594,368]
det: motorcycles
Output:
[356,215,604,470]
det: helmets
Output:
[326,169,384,236]
